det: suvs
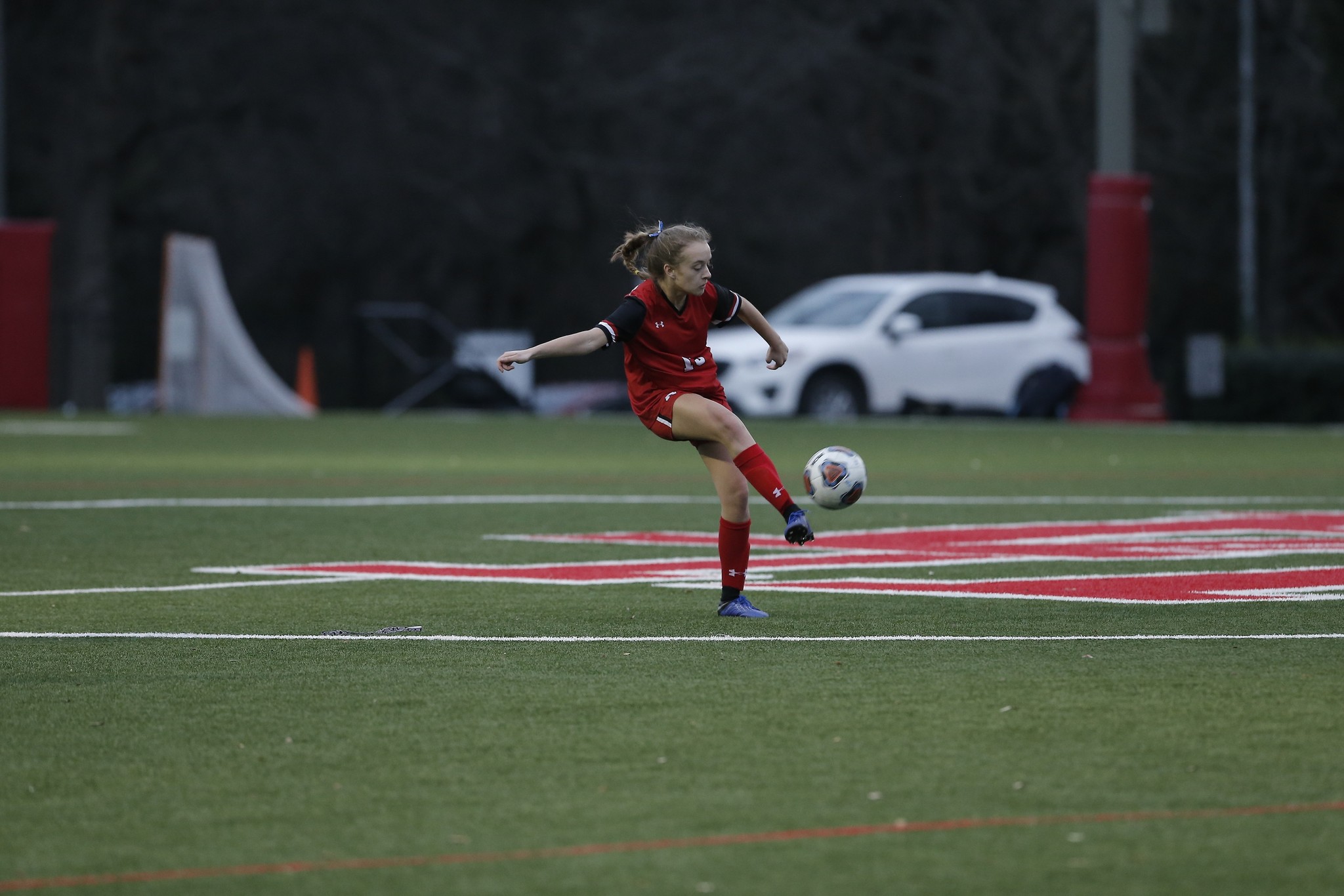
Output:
[708,271,1094,422]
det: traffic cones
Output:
[295,347,320,407]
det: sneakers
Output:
[784,509,814,546]
[717,595,769,618]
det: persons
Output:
[497,221,815,620]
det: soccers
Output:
[802,445,867,511]
